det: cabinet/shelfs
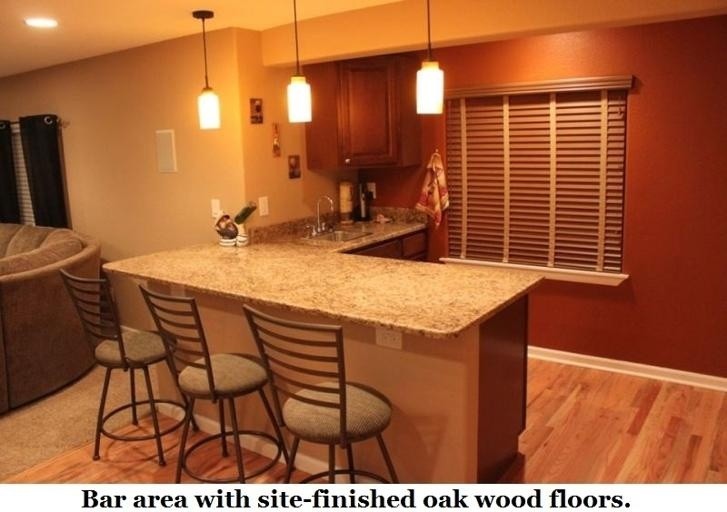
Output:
[304,52,422,175]
[342,232,428,264]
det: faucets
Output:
[315,194,333,234]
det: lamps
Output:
[413,2,445,118]
[191,9,221,133]
[281,2,316,129]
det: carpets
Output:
[1,330,163,482]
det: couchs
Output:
[0,221,106,416]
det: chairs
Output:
[241,302,401,485]
[58,269,198,469]
[135,281,294,482]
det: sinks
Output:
[304,227,374,243]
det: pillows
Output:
[0,222,87,276]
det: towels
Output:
[416,147,449,228]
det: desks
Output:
[100,215,544,481]
